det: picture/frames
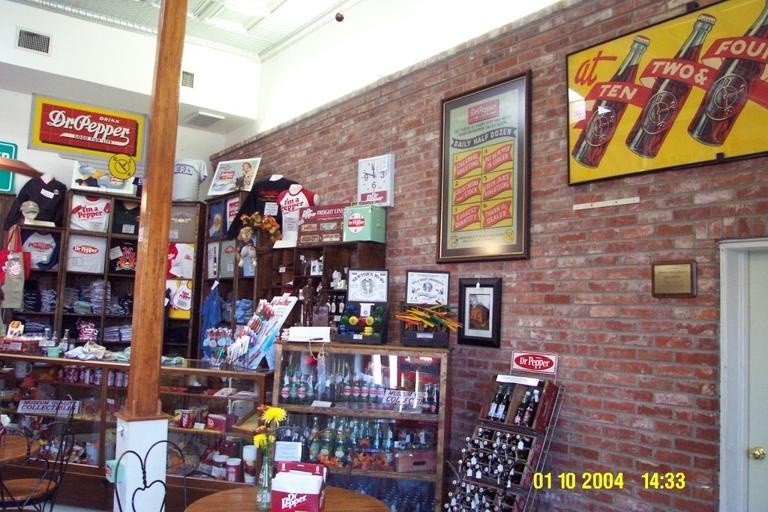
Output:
[436,1,768,348]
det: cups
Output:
[14,360,32,378]
[7,424,18,440]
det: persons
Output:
[470,295,489,330]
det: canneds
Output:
[175,405,208,428]
[212,454,229,480]
[226,458,242,481]
[63,365,128,388]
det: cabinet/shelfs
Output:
[0,189,448,512]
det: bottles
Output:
[326,294,345,314]
[280,352,440,512]
[49,440,59,458]
[329,322,337,340]
[444,382,540,511]
[257,450,271,510]
[570,1,768,171]
[0,389,20,402]
[0,327,69,355]
